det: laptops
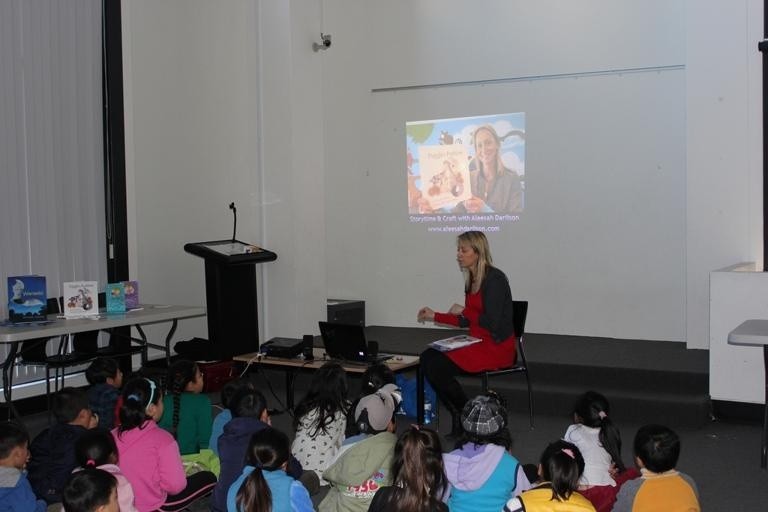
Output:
[319,321,394,365]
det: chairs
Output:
[7,291,149,424]
[477,301,535,432]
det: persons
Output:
[564,391,641,511]
[418,230,516,440]
[291,357,450,511]
[502,439,597,512]
[437,388,532,511]
[611,424,700,510]
[450,125,522,213]
[2,358,321,512]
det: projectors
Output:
[260,337,303,359]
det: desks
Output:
[232,348,425,426]
[727,319,768,469]
[0,303,206,428]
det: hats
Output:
[458,386,510,438]
[351,379,404,431]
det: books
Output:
[433,334,482,351]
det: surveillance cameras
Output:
[322,35,331,50]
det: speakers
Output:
[368,341,378,357]
[303,335,314,364]
